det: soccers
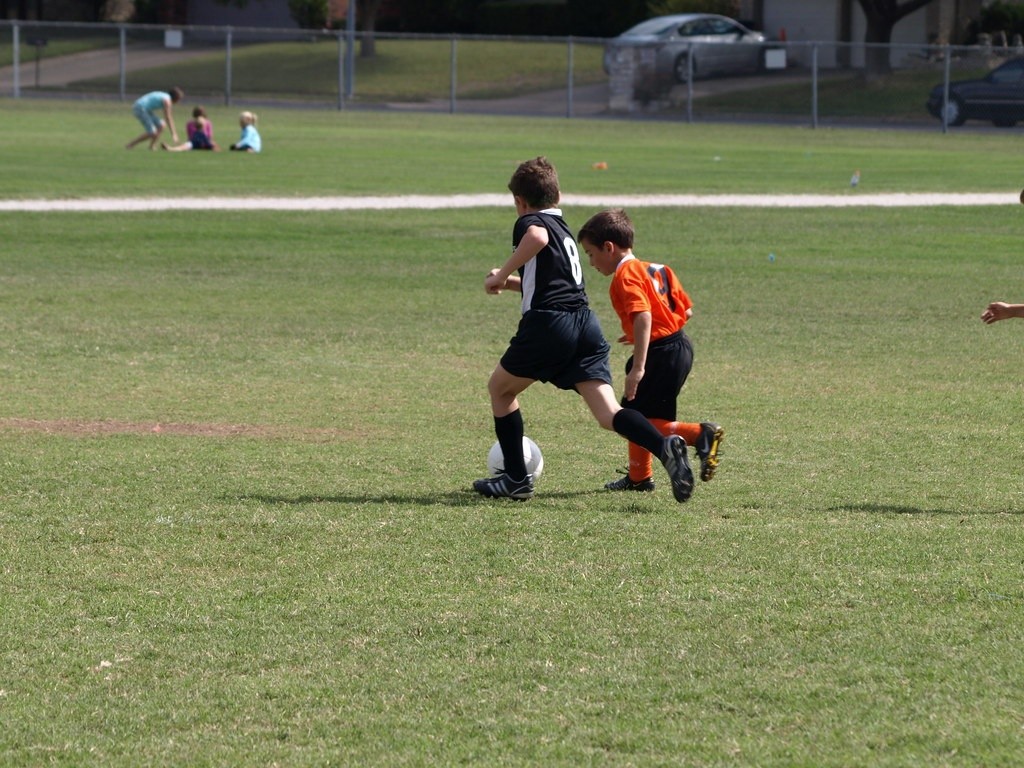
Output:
[488,435,546,484]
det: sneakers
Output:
[604,469,655,492]
[472,467,534,500]
[661,435,694,503]
[695,421,725,481]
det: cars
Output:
[927,55,1024,126]
[603,11,771,83]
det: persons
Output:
[577,207,723,494]
[980,190,1024,324]
[229,111,261,153]
[472,159,695,504]
[124,86,184,151]
[327,0,356,42]
[162,105,220,153]
